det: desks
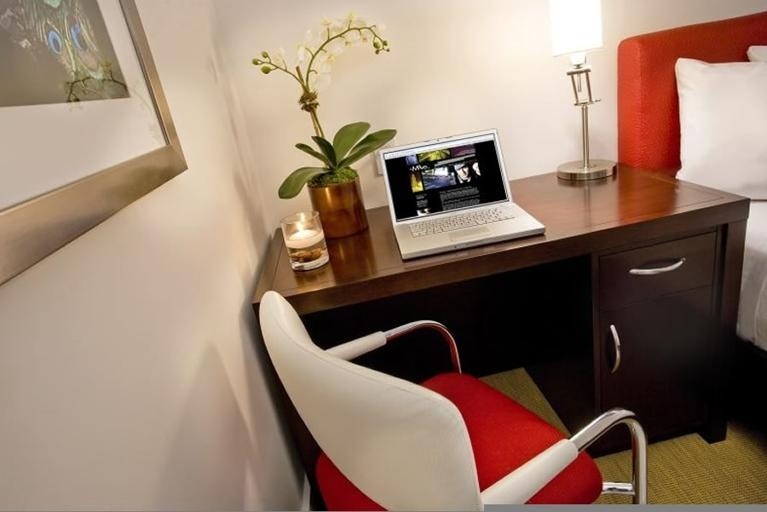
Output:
[252,167,751,445]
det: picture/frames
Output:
[0,0,187,287]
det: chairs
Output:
[260,290,647,511]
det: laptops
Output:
[378,127,546,259]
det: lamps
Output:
[546,0,618,180]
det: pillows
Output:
[675,43,767,201]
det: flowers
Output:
[251,12,391,198]
[61,47,129,105]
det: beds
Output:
[619,11,767,448]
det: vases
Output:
[307,173,368,238]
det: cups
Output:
[279,210,331,272]
